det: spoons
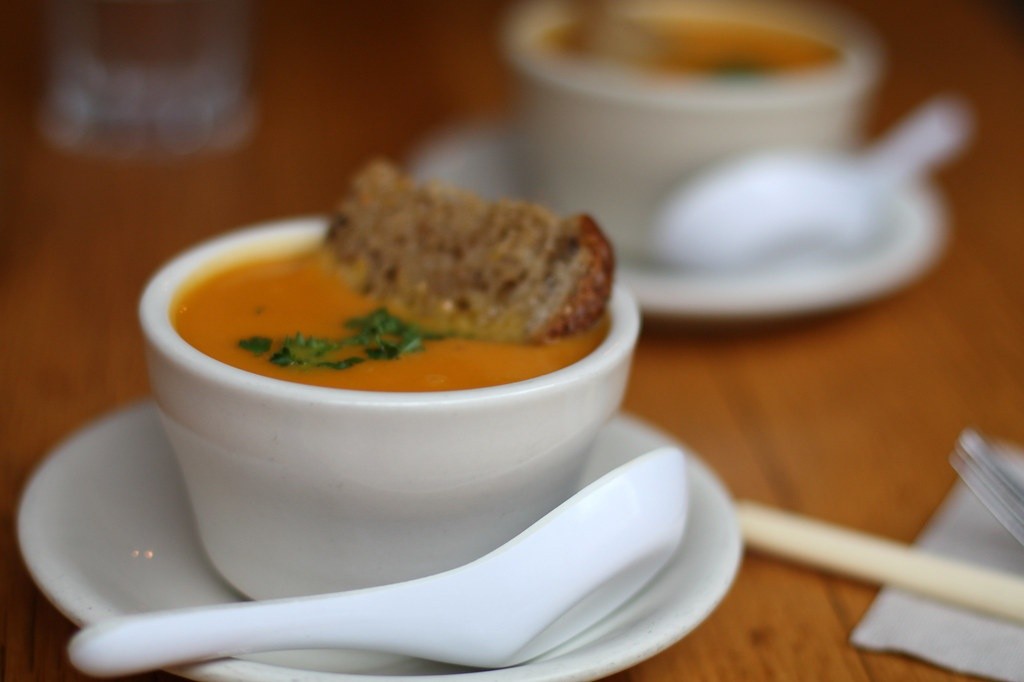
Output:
[67,444,690,681]
[647,102,972,278]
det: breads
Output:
[322,158,616,344]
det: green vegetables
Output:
[236,307,450,372]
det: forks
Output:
[949,429,1023,551]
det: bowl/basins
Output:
[498,1,885,255]
[137,214,642,601]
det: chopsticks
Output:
[733,490,1024,626]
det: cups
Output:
[32,0,263,165]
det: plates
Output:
[15,394,748,682]
[411,127,949,325]
